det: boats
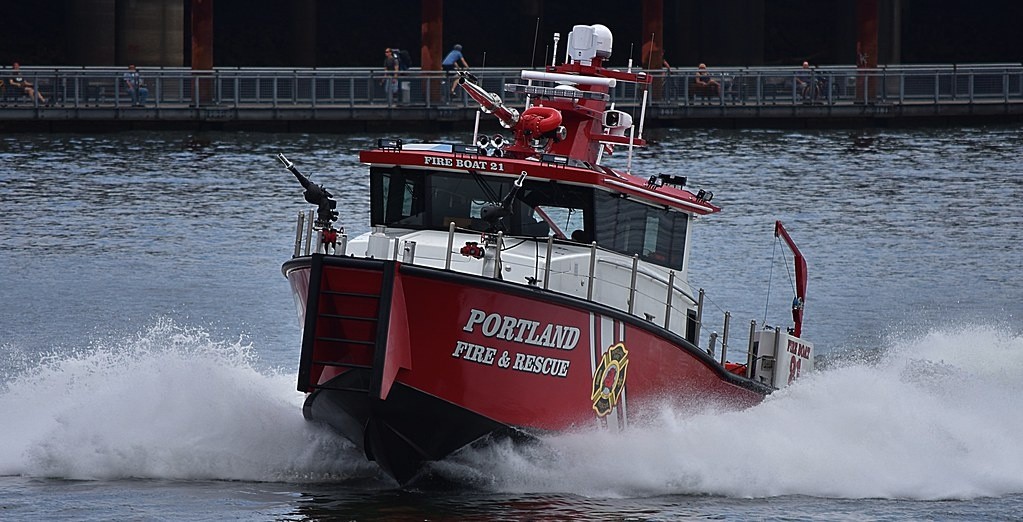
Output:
[275,23,816,494]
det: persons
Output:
[8,63,46,103]
[696,64,726,105]
[124,64,148,107]
[379,47,398,106]
[796,62,818,97]
[441,44,469,96]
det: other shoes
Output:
[451,91,457,96]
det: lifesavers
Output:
[522,106,563,133]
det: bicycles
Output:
[438,65,473,103]
[659,57,678,102]
[803,74,841,105]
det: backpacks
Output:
[389,49,412,72]
